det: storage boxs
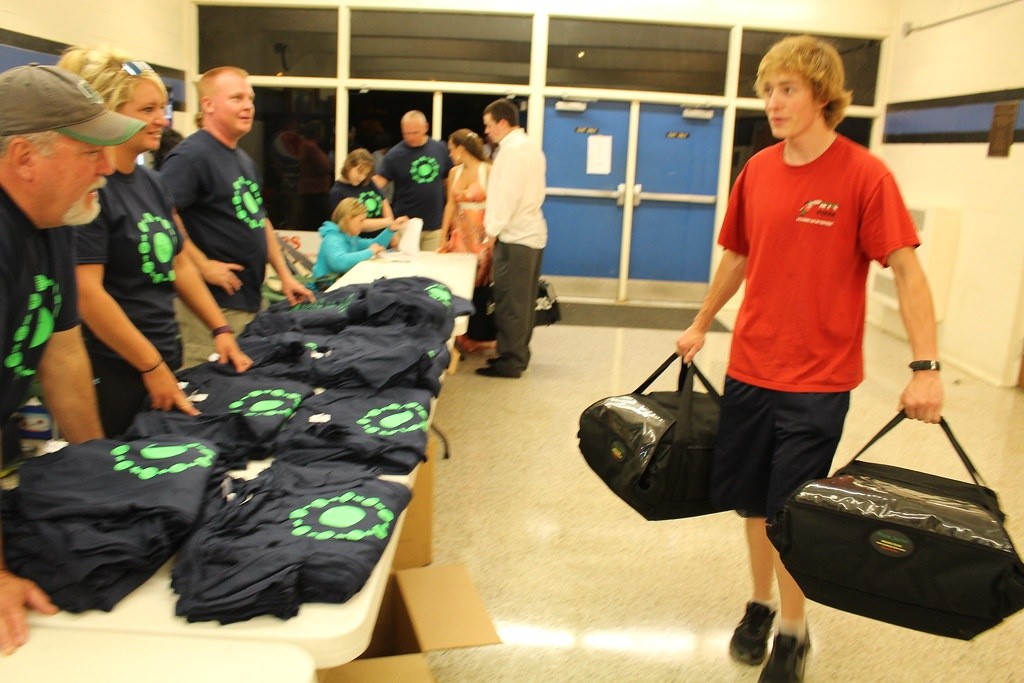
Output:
[317,444,501,682]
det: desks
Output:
[1,250,478,681]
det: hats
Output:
[0,61,148,147]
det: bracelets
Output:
[910,360,940,371]
[141,359,165,373]
[212,325,234,338]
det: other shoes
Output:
[487,355,509,365]
[474,366,521,379]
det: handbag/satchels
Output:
[766,404,1024,640]
[580,343,728,520]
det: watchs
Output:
[479,236,489,244]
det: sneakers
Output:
[757,621,815,680]
[729,596,777,667]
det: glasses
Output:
[116,63,141,78]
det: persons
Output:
[437,128,497,288]
[57,44,253,438]
[160,65,315,370]
[675,35,943,683]
[328,147,395,240]
[0,62,149,656]
[370,110,453,251]
[474,99,548,378]
[151,127,183,167]
[312,196,408,292]
